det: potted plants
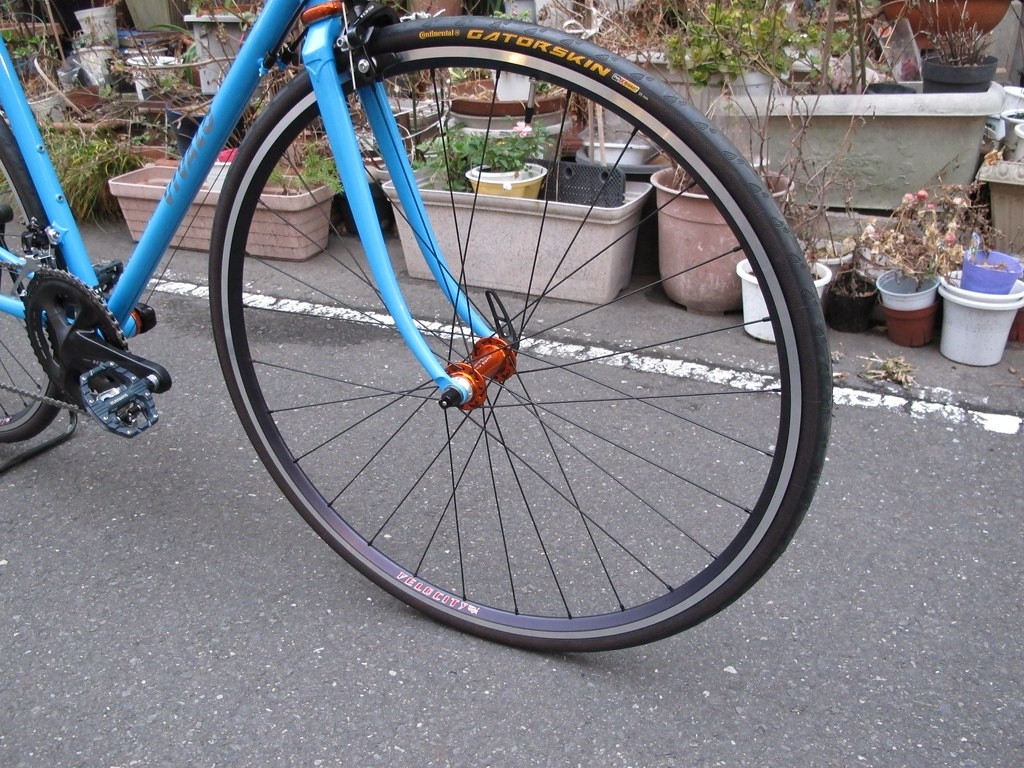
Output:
[0,0,1024,368]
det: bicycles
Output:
[0,1,834,659]
